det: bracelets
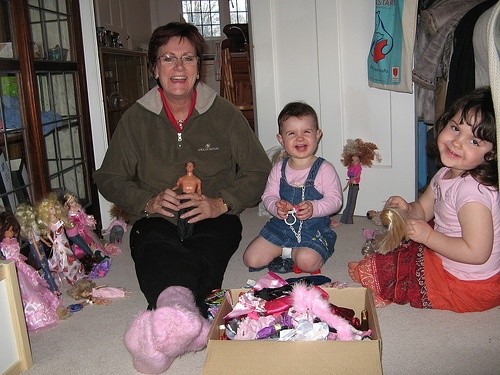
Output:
[143,202,150,218]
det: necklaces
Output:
[169,109,189,127]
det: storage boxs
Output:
[202,287,384,375]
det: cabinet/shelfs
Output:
[0,0,103,235]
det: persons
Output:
[171,161,201,242]
[340,156,362,224]
[95,22,272,375]
[367,209,416,255]
[243,102,344,272]
[0,191,127,333]
[348,86,500,312]
